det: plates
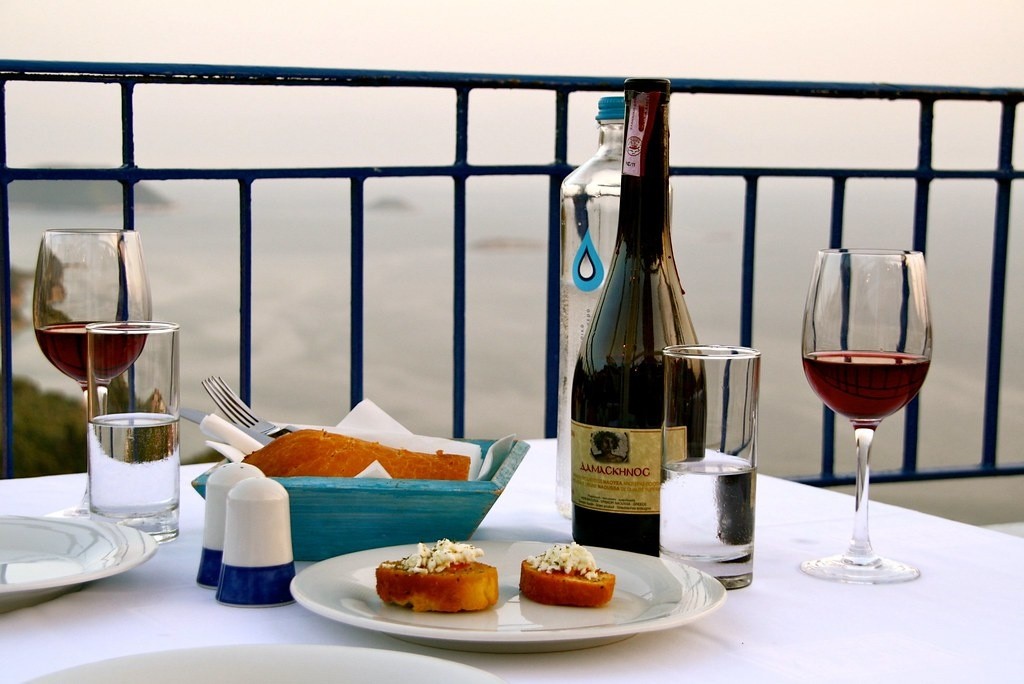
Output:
[0,514,158,618]
[289,542,727,654]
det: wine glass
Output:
[800,247,933,587]
[32,228,152,517]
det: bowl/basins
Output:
[192,432,531,567]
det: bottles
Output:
[559,79,707,556]
[195,461,297,607]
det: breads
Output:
[520,560,615,607]
[375,557,499,612]
[240,429,471,481]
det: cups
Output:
[661,344,761,589]
[87,320,180,544]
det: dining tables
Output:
[0,440,1024,684]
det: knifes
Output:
[166,403,275,449]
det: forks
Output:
[198,374,299,437]
